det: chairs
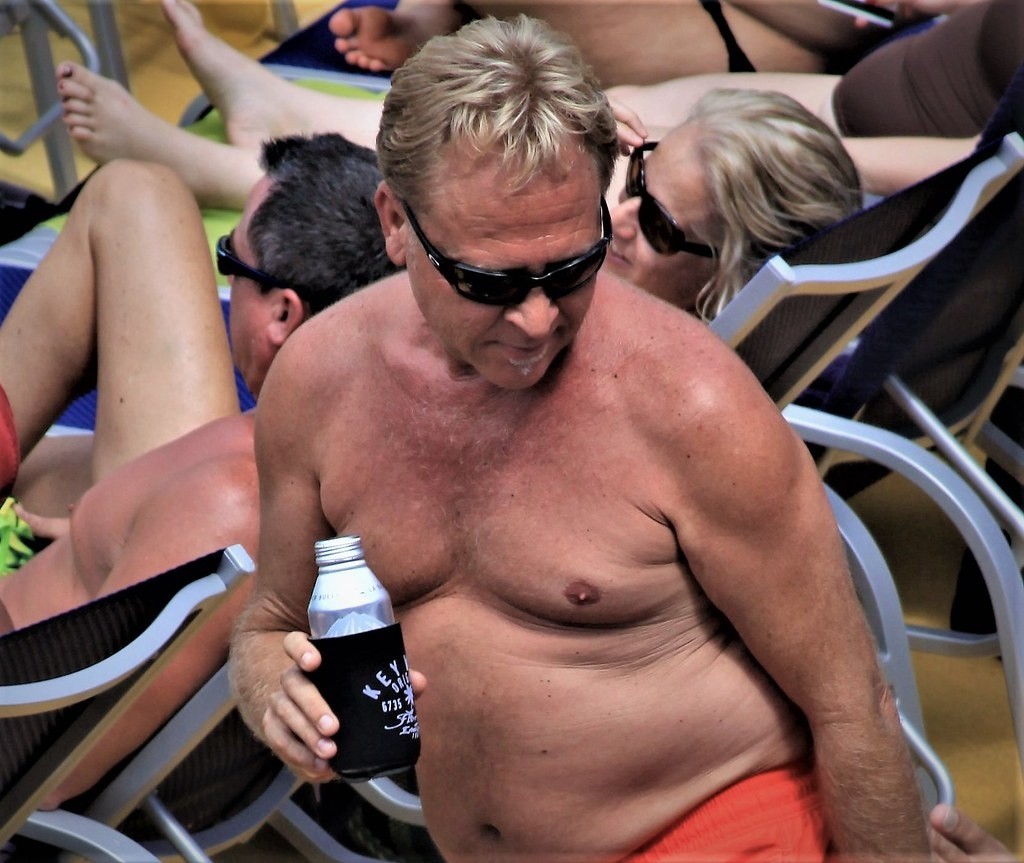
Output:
[0,0,1023,863]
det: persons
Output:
[1,0,1024,863]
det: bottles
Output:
[307,532,421,779]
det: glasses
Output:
[400,194,613,307]
[217,235,294,288]
[626,140,719,259]
